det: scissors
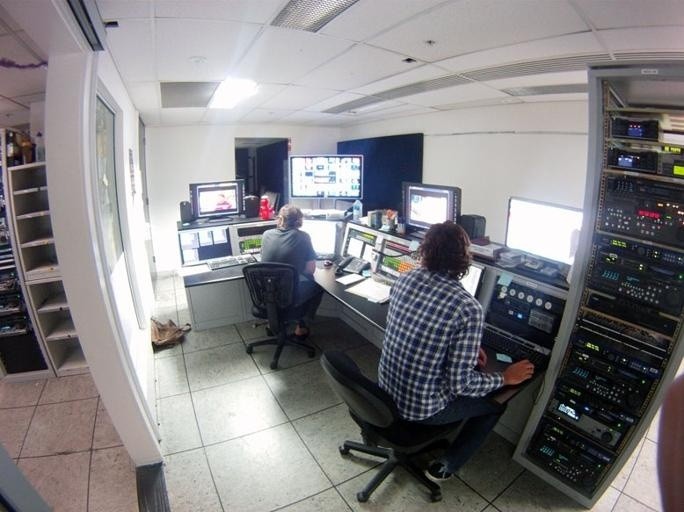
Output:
[386,209,394,218]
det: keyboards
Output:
[206,254,257,270]
[480,328,544,365]
[316,255,334,260]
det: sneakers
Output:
[424,457,453,483]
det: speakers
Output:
[244,195,259,218]
[180,201,191,226]
[458,214,486,241]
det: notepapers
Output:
[362,270,370,277]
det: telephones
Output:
[338,256,370,274]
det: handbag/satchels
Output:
[151,318,192,345]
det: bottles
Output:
[7,129,45,166]
[259,195,270,220]
[352,199,363,221]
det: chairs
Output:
[321,349,467,503]
[241,262,317,371]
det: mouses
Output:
[324,260,333,266]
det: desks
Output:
[183,260,551,410]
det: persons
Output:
[260,204,323,337]
[217,194,231,209]
[377,220,534,482]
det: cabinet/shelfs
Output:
[0,128,92,382]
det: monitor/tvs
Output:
[505,196,583,278]
[288,154,364,200]
[400,181,461,239]
[189,179,245,223]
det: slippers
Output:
[294,326,310,340]
